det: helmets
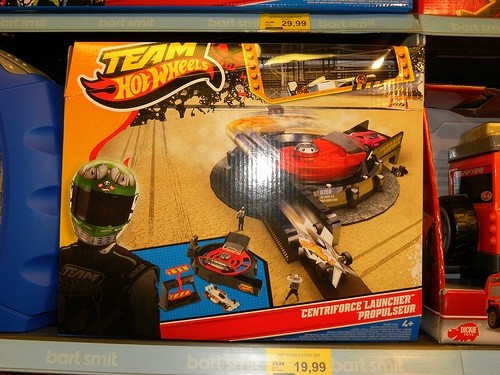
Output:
[68,158,139,245]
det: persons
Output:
[60,157,161,335]
